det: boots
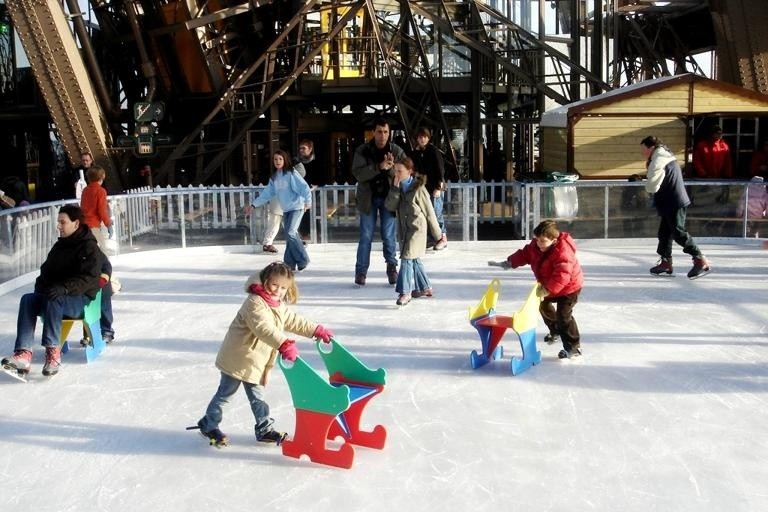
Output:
[688,257,709,277]
[650,257,672,274]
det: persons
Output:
[385,158,443,305]
[640,136,711,280]
[244,140,325,271]
[198,261,334,440]
[505,220,583,358]
[0,206,103,383]
[620,174,650,238]
[0,153,119,347]
[412,128,448,250]
[693,127,768,238]
[352,119,408,288]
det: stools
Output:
[278,337,386,469]
[467,278,544,375]
[40,287,107,364]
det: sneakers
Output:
[198,416,228,445]
[558,348,582,359]
[544,333,561,342]
[80,335,114,347]
[355,272,366,285]
[263,245,279,252]
[386,264,432,305]
[427,233,447,249]
[1,350,31,374]
[257,429,290,442]
[42,347,61,375]
[287,265,306,270]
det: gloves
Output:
[314,324,334,343]
[108,227,113,239]
[46,286,68,300]
[279,339,298,361]
[98,273,109,288]
